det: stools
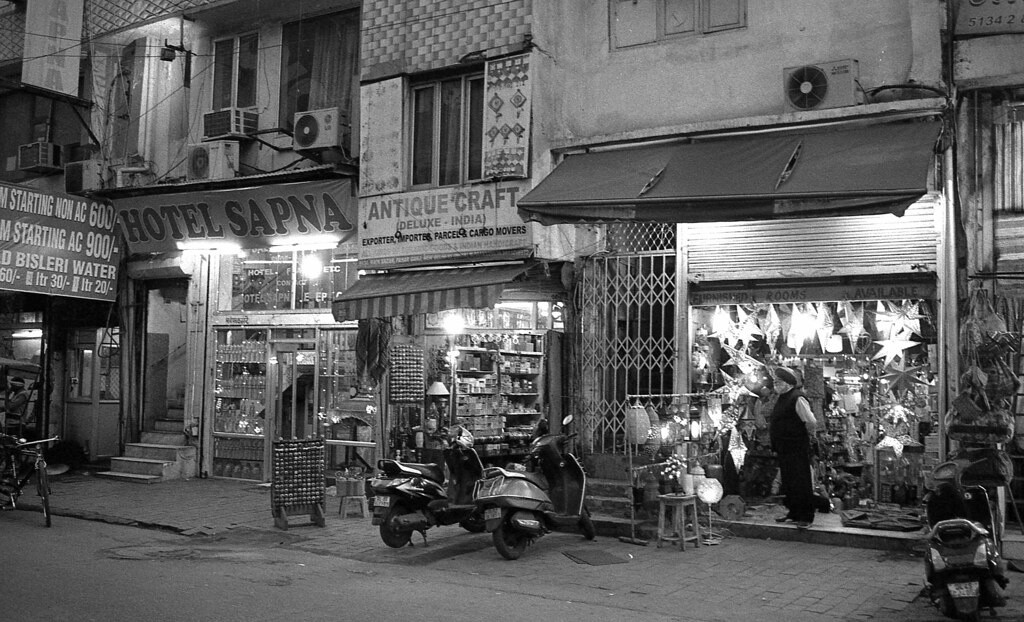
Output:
[338,495,370,519]
[659,491,702,552]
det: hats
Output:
[775,368,798,385]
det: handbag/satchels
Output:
[939,287,1023,487]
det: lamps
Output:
[426,381,450,433]
[160,38,197,62]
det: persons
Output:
[5,376,29,471]
[769,368,817,529]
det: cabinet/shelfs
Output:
[211,361,266,477]
[421,305,545,458]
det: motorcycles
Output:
[915,449,1012,622]
[366,424,489,548]
[471,415,599,556]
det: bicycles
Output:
[1,433,58,526]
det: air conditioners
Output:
[783,55,860,116]
[293,107,346,152]
[186,107,258,181]
[64,160,108,194]
[18,143,62,176]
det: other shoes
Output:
[798,521,812,528]
[776,515,798,523]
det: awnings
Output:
[515,121,943,226]
[331,265,534,322]
